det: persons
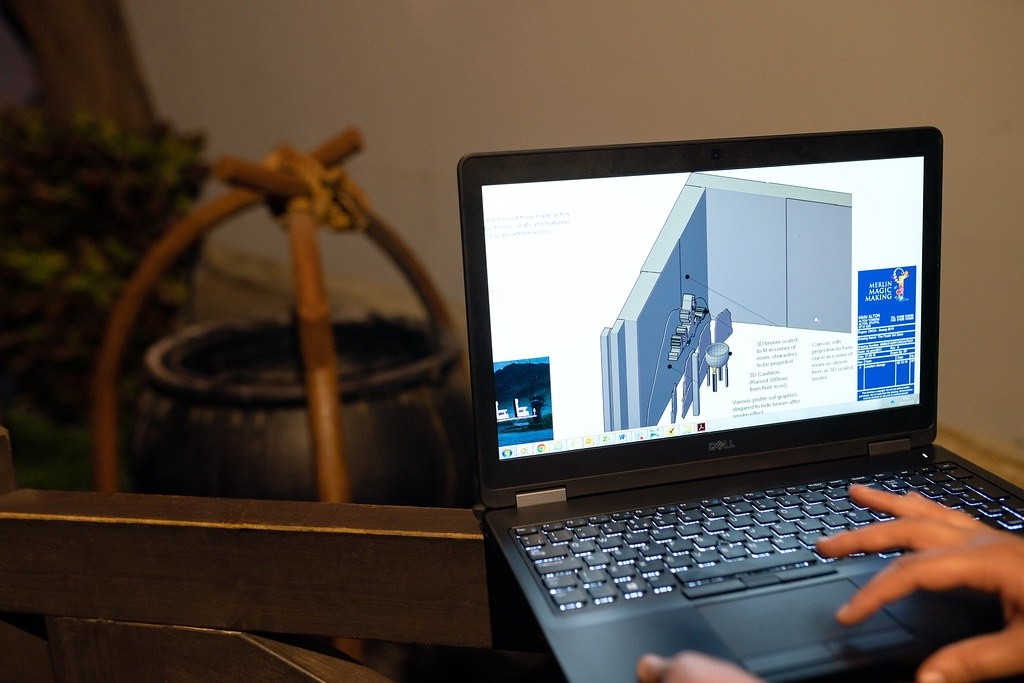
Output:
[636,484,1024,683]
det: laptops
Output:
[457,125,1024,683]
